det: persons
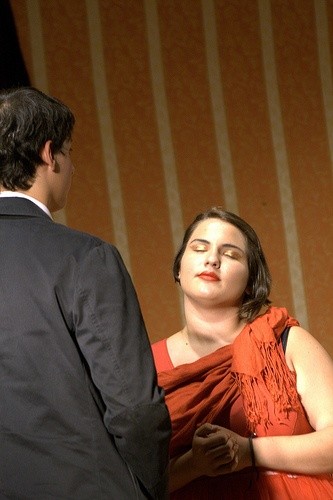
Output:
[0,84,175,499]
[147,207,333,499]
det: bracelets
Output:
[247,435,258,472]
